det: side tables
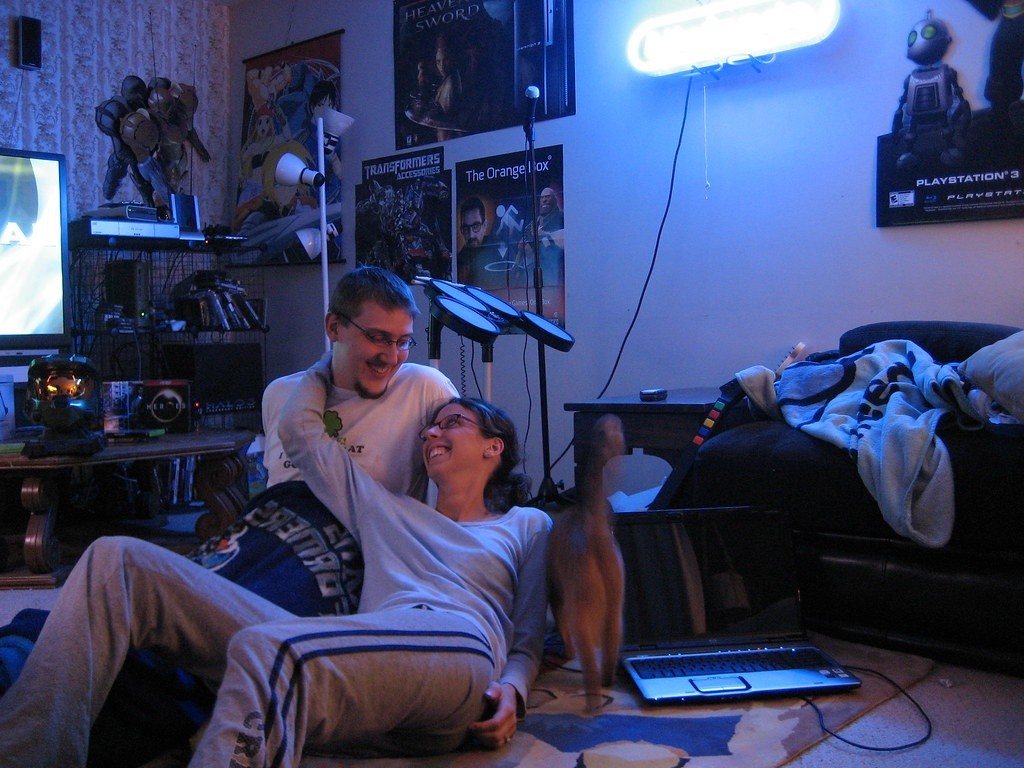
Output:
[563,386,722,487]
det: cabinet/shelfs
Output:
[68,236,270,515]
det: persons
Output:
[1,264,462,697]
[1,348,553,768]
[405,29,565,290]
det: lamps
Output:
[324,107,354,154]
[274,151,324,188]
[296,222,343,259]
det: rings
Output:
[505,737,512,743]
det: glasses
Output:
[342,314,418,352]
[459,219,484,235]
[418,412,488,440]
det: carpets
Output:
[283,630,935,768]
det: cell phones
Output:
[640,389,667,401]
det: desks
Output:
[0,429,257,591]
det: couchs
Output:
[690,322,1024,679]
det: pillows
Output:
[957,329,1024,422]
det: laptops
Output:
[605,504,862,706]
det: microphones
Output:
[523,86,539,131]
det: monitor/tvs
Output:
[0,146,72,357]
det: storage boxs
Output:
[95,379,201,433]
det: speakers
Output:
[105,260,150,333]
[171,194,201,233]
